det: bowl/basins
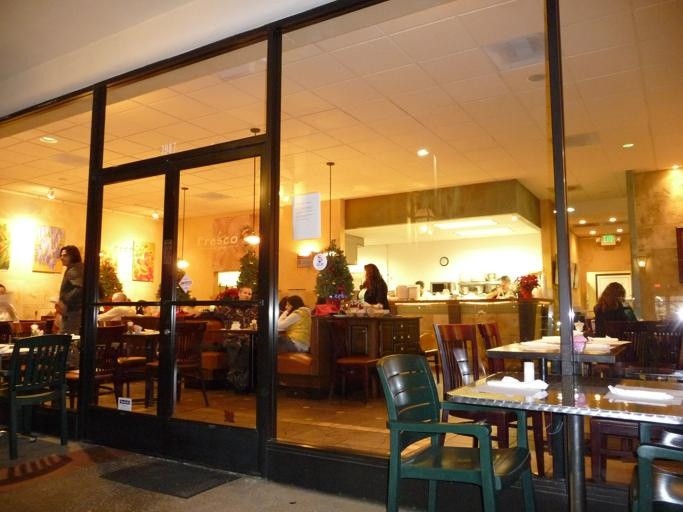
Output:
[366,310,384,318]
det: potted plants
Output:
[314,239,354,308]
[98,260,123,310]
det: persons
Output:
[0,282,20,326]
[97,292,157,321]
[55,246,85,343]
[211,287,312,395]
[488,275,512,302]
[415,279,434,300]
[592,283,627,338]
[356,263,393,317]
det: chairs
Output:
[577,319,683,482]
[327,321,379,401]
[624,423,683,511]
[430,320,553,478]
[376,354,539,512]
[1,321,210,459]
[485,335,633,373]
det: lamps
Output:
[244,128,259,245]
[324,162,337,255]
[177,187,190,268]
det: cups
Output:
[249,323,256,330]
[232,321,240,330]
[134,327,141,335]
[522,361,534,383]
[127,321,134,335]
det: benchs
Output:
[278,317,330,397]
[184,319,229,387]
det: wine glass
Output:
[574,321,583,336]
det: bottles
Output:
[31,325,37,335]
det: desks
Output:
[445,371,682,510]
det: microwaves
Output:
[429,282,456,295]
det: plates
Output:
[396,285,419,300]
